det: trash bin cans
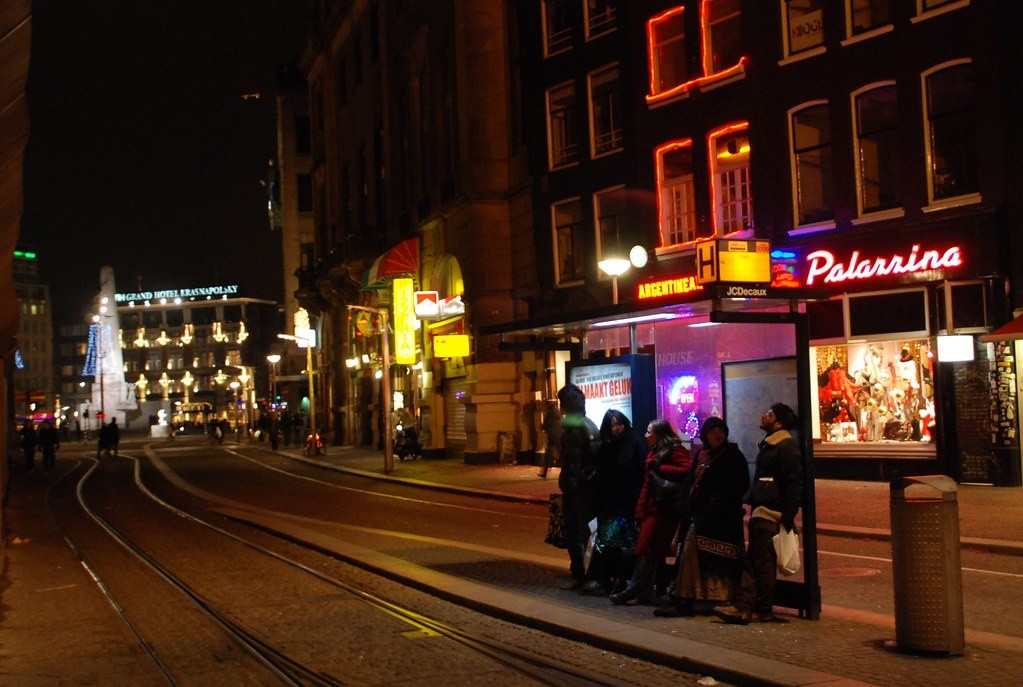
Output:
[889,474,966,657]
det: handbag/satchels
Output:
[544,492,568,548]
[772,521,801,575]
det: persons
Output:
[654,415,751,617]
[207,409,325,453]
[609,419,690,608]
[19,416,120,471]
[712,402,804,625]
[583,409,649,595]
[388,402,413,446]
[537,400,563,478]
[558,384,597,591]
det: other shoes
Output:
[536,472,547,479]
[652,600,697,618]
[560,576,586,590]
[608,584,648,604]
[712,604,751,623]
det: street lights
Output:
[266,355,281,404]
[597,258,631,356]
[277,333,316,457]
[238,376,250,439]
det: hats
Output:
[699,416,729,442]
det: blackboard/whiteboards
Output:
[496,430,518,465]
